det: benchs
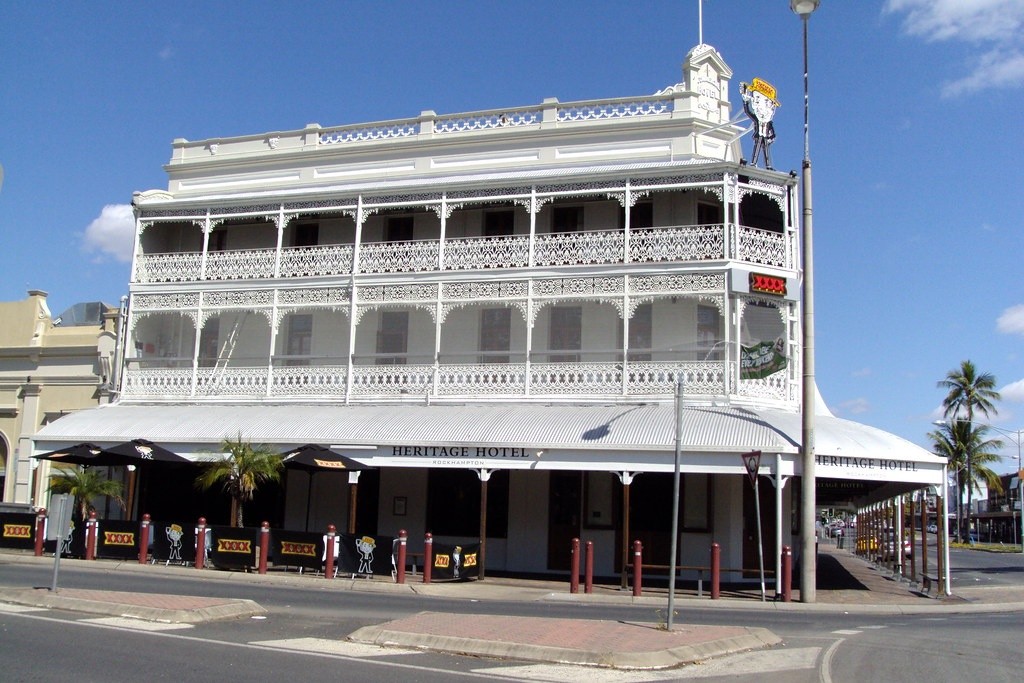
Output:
[393,553,425,575]
[893,563,908,581]
[874,555,885,571]
[919,572,945,597]
[627,563,776,596]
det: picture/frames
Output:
[393,496,407,516]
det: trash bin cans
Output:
[837,538,845,549]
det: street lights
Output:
[790,0,819,607]
[932,420,1024,554]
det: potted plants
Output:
[189,435,287,570]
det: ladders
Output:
[202,312,250,395]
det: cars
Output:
[873,532,912,561]
[828,527,844,538]
[928,525,939,534]
[853,535,879,553]
[953,529,968,538]
[969,528,985,540]
